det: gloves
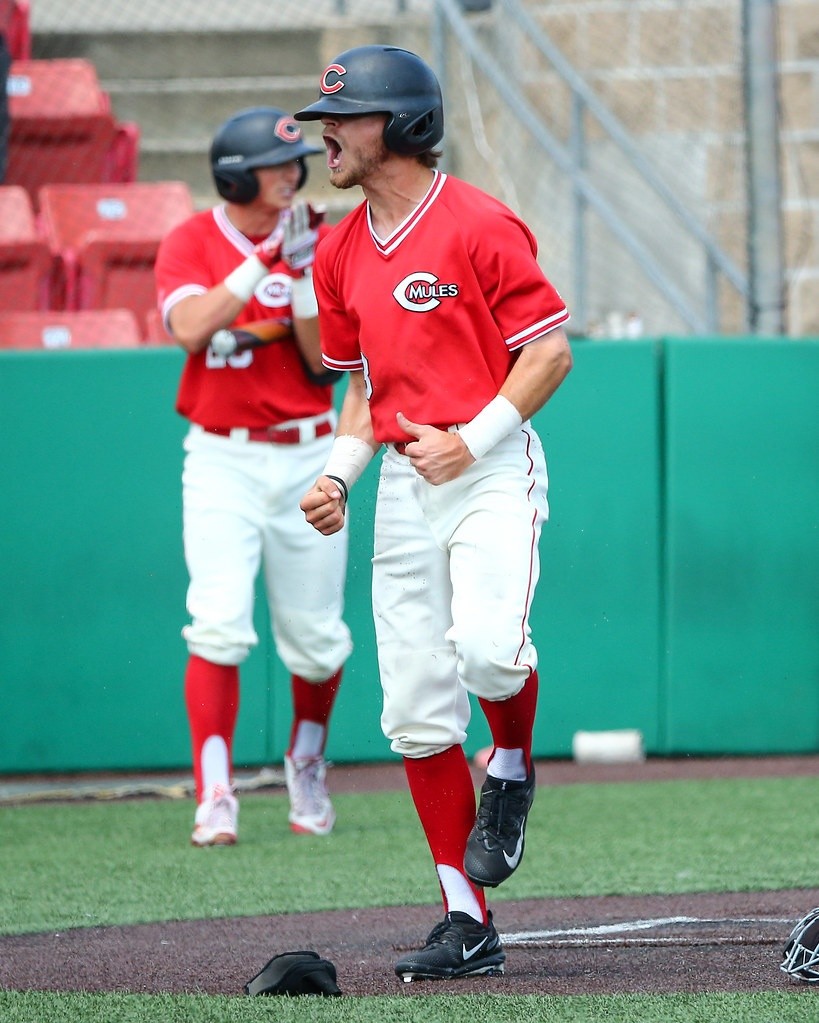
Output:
[253,210,285,273]
[283,200,325,279]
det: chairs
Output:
[0,0,194,348]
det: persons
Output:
[155,109,354,846]
[294,45,574,982]
[0,31,10,185]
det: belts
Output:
[393,427,448,455]
[204,421,332,444]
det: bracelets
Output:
[453,395,525,462]
[318,436,373,505]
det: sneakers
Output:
[394,909,506,983]
[284,755,336,837]
[191,782,241,846]
[463,757,536,888]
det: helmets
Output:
[209,108,323,203]
[293,45,444,155]
